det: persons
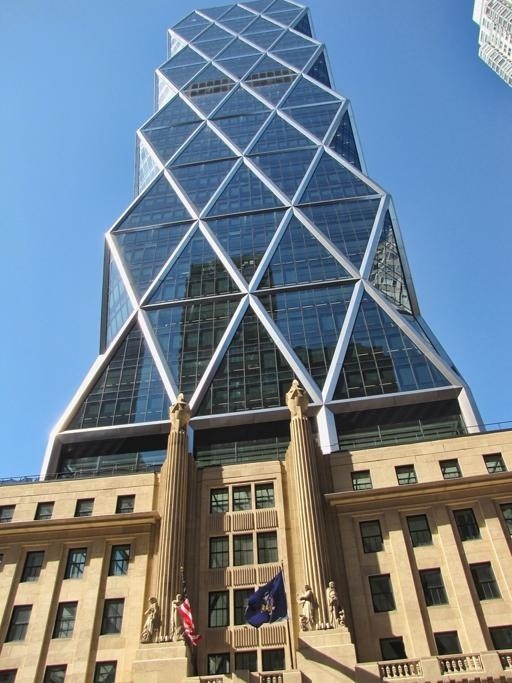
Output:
[172,594,184,636]
[144,597,157,635]
[296,585,317,630]
[326,581,339,628]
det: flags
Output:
[178,598,204,647]
[245,570,287,628]
